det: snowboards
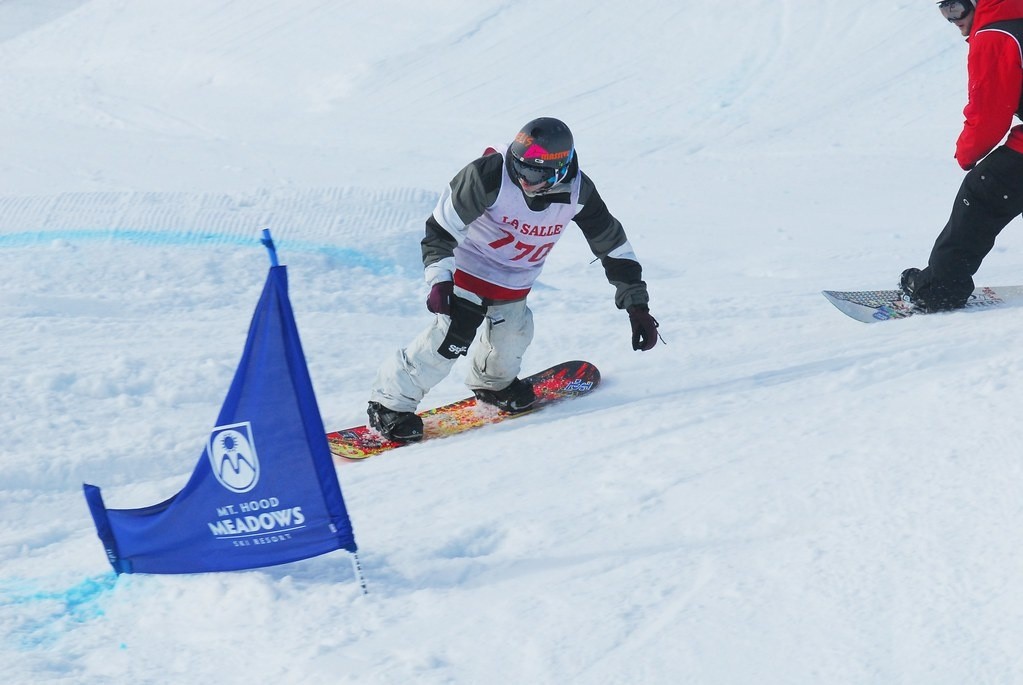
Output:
[821,284,1023,323]
[324,360,602,460]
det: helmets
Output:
[511,117,574,168]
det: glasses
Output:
[939,1,970,23]
[512,156,554,186]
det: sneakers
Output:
[473,377,538,410]
[368,401,423,442]
[901,268,964,311]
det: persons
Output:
[900,0,1023,309]
[369,116,660,442]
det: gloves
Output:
[628,307,659,352]
[426,279,454,316]
